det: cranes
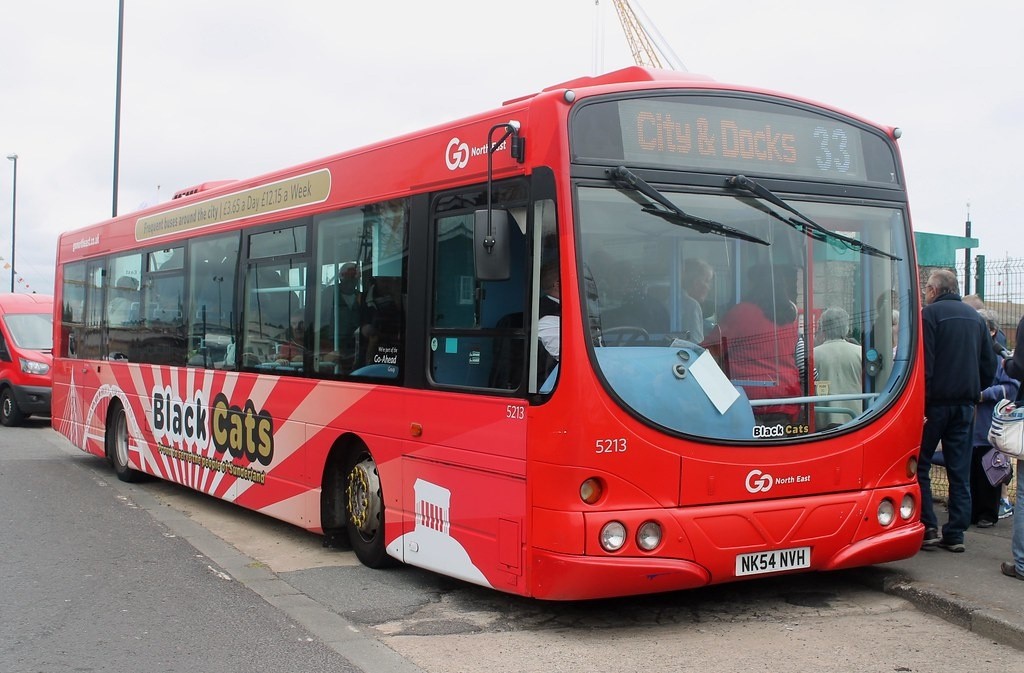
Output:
[612,0,663,72]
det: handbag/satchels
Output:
[988,398,1024,461]
[982,448,1012,486]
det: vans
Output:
[1,294,55,427]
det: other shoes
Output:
[1001,561,1023,580]
[977,520,993,526]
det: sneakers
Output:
[923,528,939,545]
[998,499,1013,517]
[939,538,965,552]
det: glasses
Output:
[921,284,933,294]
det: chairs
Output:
[129,301,285,369]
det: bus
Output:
[50,71,924,604]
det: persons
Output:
[698,260,800,437]
[796,290,1013,528]
[102,242,403,378]
[922,270,998,552]
[1001,313,1024,580]
[523,247,716,396]
